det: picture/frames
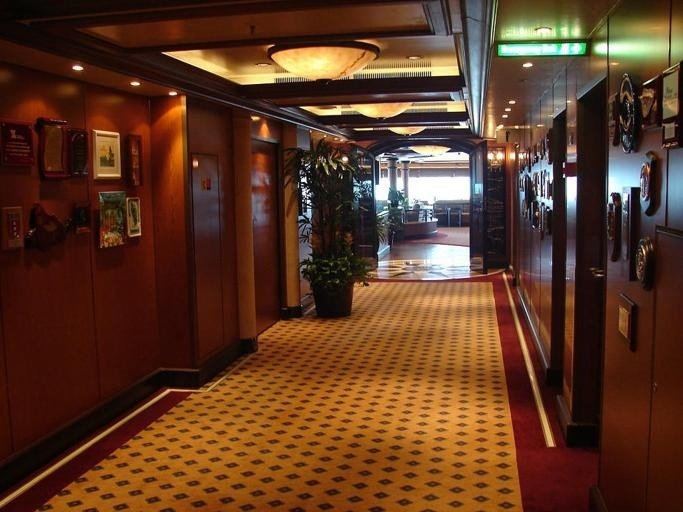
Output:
[636,60,682,152]
[89,127,144,250]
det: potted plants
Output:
[278,139,375,319]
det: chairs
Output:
[432,199,470,227]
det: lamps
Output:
[265,39,382,85]
